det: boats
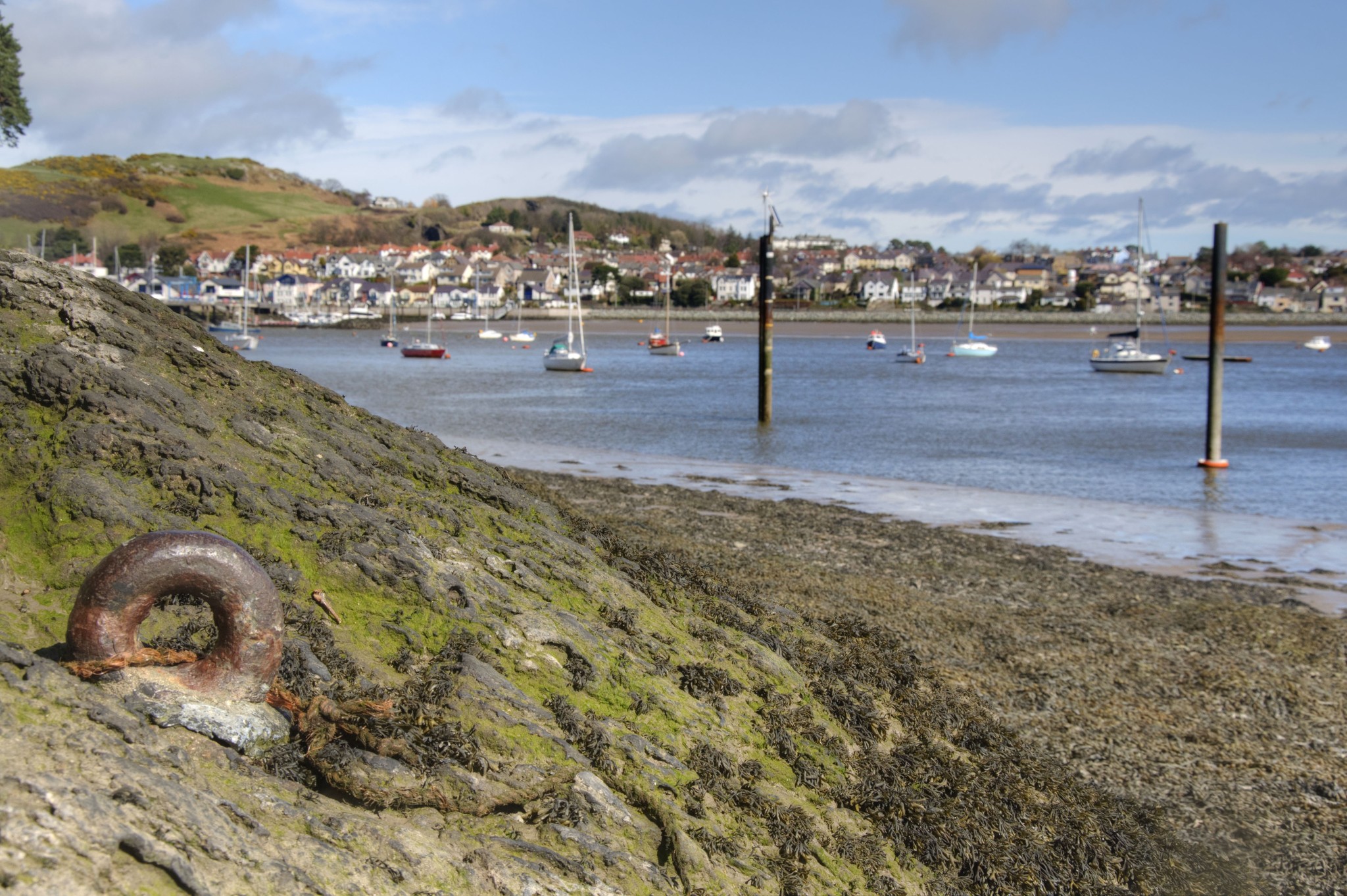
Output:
[430,310,447,322]
[700,322,725,343]
[449,312,476,322]
[865,326,885,351]
[1303,333,1334,353]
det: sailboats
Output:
[1088,193,1185,375]
[399,256,451,359]
[507,295,537,343]
[646,262,684,357]
[947,260,999,360]
[199,241,263,352]
[543,211,594,373]
[379,270,400,348]
[477,285,504,339]
[895,271,927,365]
